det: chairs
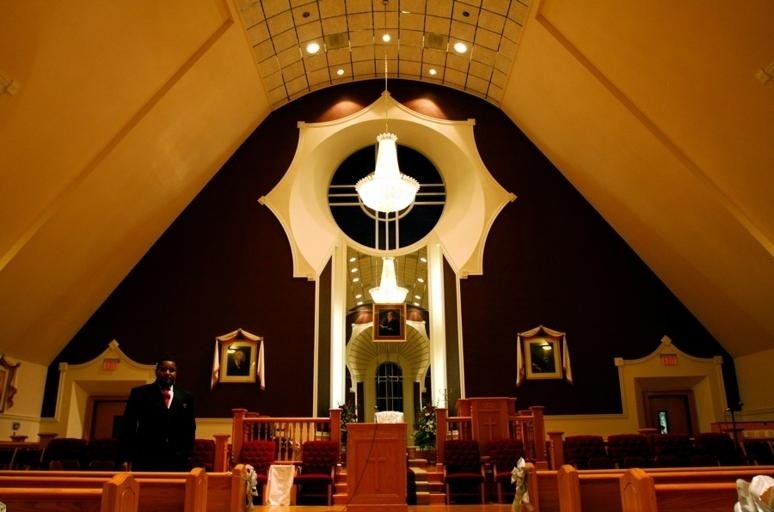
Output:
[0,438,215,472]
[293,440,339,506]
[442,439,525,505]
[564,432,774,469]
[237,439,278,505]
[512,457,774,512]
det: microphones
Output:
[373,405,378,409]
[724,401,744,412]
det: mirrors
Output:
[346,245,432,446]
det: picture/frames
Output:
[217,339,259,385]
[0,362,12,413]
[372,303,406,343]
[521,336,565,381]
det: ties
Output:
[161,387,170,408]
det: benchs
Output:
[0,467,245,512]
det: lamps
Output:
[369,257,409,304]
[354,0,421,212]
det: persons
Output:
[227,350,249,376]
[113,360,196,471]
[379,309,400,335]
[532,348,555,372]
